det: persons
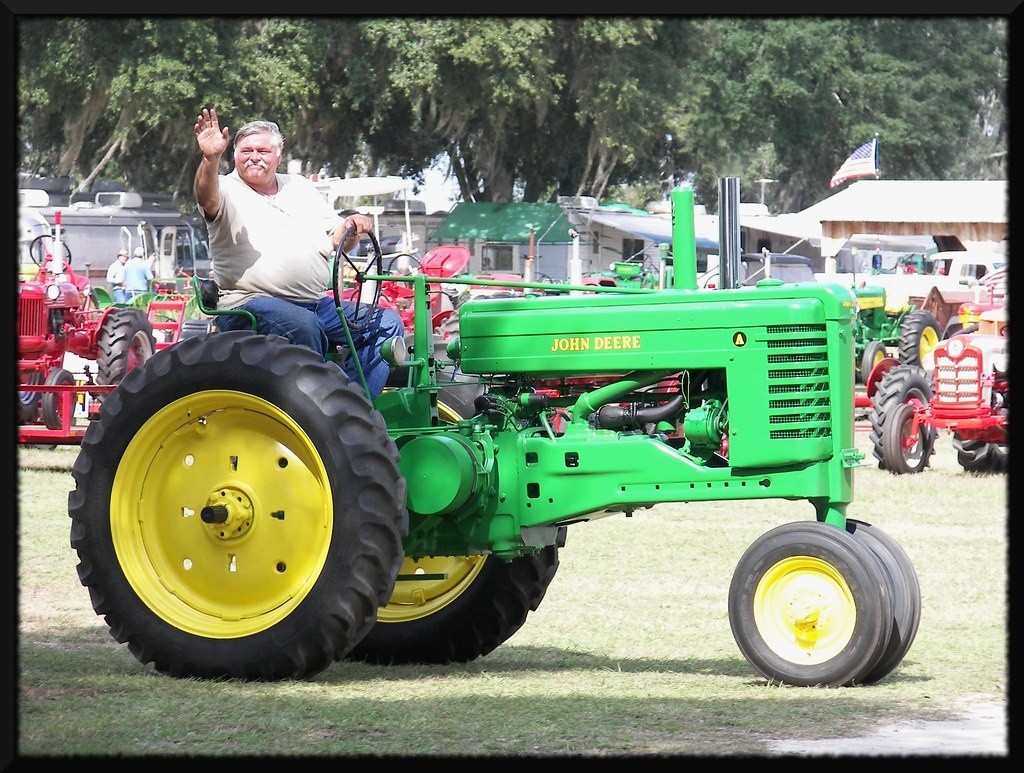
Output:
[194,107,406,405]
[106,248,130,304]
[122,247,155,305]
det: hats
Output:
[134,247,144,256]
[118,248,130,258]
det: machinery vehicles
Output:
[17,198,1011,475]
[66,176,923,690]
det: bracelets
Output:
[349,232,362,239]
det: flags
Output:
[829,135,877,187]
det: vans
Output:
[119,218,210,295]
